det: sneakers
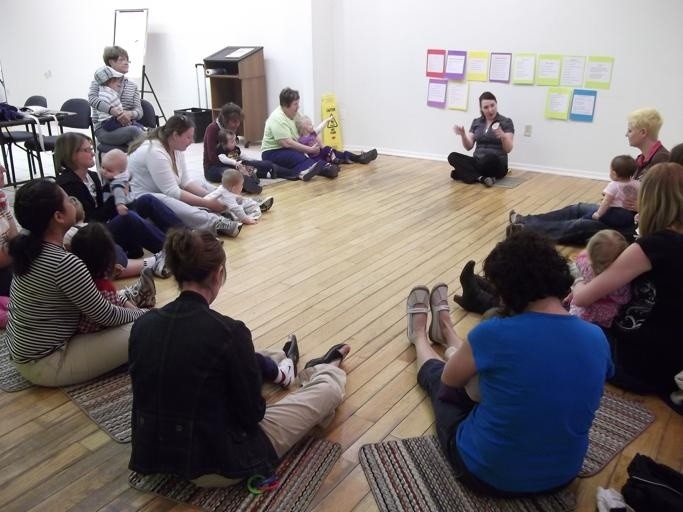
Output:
[126,266,158,309]
[450,170,460,181]
[320,164,341,179]
[357,149,378,165]
[428,283,450,346]
[480,176,496,188]
[299,160,324,182]
[248,196,275,213]
[405,284,431,346]
[278,356,295,388]
[151,248,172,279]
[214,219,244,238]
[242,175,262,195]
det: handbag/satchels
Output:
[0,103,24,122]
[19,105,54,118]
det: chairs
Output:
[97,99,157,166]
[24,98,96,180]
[0,95,47,188]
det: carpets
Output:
[476,178,527,188]
[357,435,579,511]
[574,388,657,477]
[257,177,286,186]
[59,362,133,444]
[0,328,37,393]
[128,437,342,512]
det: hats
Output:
[94,66,125,86]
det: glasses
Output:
[73,148,95,154]
[117,60,132,64]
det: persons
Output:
[408,230,615,499]
[261,86,376,178]
[88,48,147,149]
[127,228,350,487]
[448,91,514,187]
[453,107,683,397]
[0,102,274,387]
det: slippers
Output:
[280,334,299,380]
[304,343,351,373]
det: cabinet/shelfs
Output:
[203,45,267,142]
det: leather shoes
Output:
[507,225,521,241]
[508,209,519,224]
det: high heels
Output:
[472,272,498,302]
[453,260,495,314]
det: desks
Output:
[0,107,78,188]
